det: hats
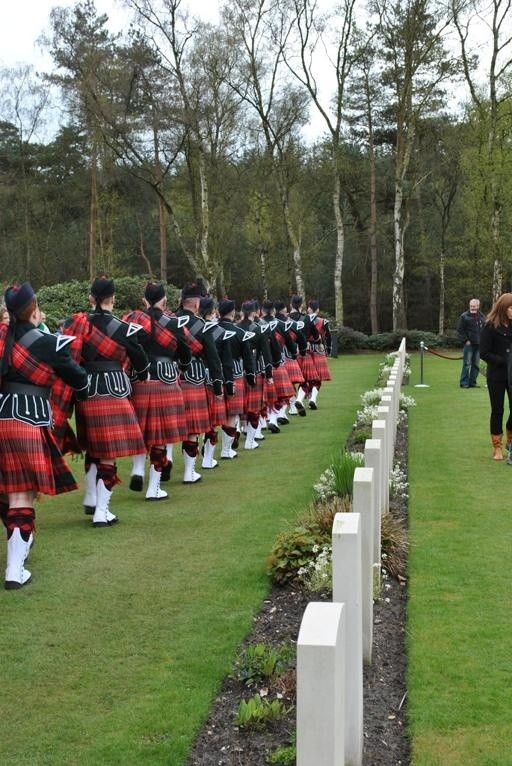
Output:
[218,298,234,311]
[4,281,34,311]
[242,300,284,310]
[200,297,213,307]
[92,276,114,294]
[293,295,319,308]
[184,281,201,294]
[146,280,164,296]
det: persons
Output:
[457,298,485,388]
[479,293,512,460]
[1,277,333,591]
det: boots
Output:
[129,443,173,501]
[220,415,241,459]
[241,412,267,450]
[491,434,504,460]
[201,438,217,469]
[309,386,318,409]
[289,387,307,416]
[83,463,119,528]
[183,449,201,483]
[268,403,289,433]
[4,527,33,590]
